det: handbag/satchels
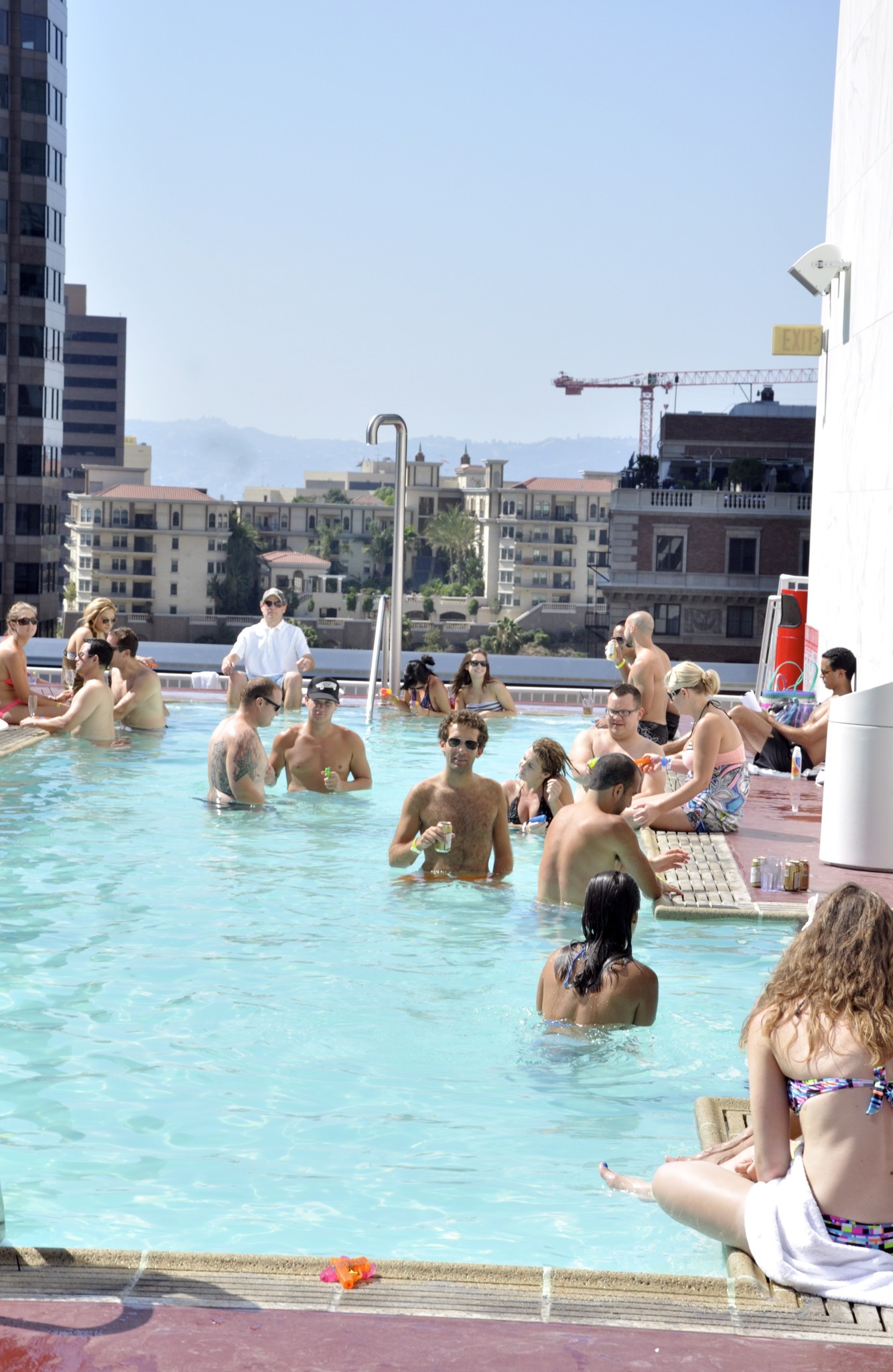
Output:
[774,697,822,728]
[760,661,818,712]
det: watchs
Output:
[410,837,422,854]
[522,821,526,833]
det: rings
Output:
[642,816,645,819]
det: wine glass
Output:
[65,668,75,701]
[28,695,37,718]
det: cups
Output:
[771,855,791,888]
[28,673,36,684]
[589,693,596,704]
[448,690,455,707]
[760,855,782,892]
[583,698,594,715]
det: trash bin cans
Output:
[818,683,891,871]
[772,588,808,690]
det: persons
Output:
[19,638,116,740]
[221,587,315,715]
[380,654,517,718]
[389,709,514,880]
[661,646,856,773]
[533,611,750,1062]
[265,676,372,794]
[62,597,170,716]
[502,737,575,835]
[106,626,166,730]
[598,882,893,1255]
[0,601,74,725]
[207,676,283,810]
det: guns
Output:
[329,1256,371,1290]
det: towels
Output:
[741,750,826,788]
[191,671,222,690]
[742,1140,893,1310]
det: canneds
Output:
[606,640,619,661]
[784,859,800,892]
[799,859,810,890]
[435,821,452,852]
[750,856,767,888]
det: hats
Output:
[307,676,339,704]
[262,588,285,603]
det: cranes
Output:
[553,368,818,456]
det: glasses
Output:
[98,618,116,624]
[606,708,639,716]
[446,738,479,751]
[469,660,486,666]
[611,636,625,645]
[13,618,37,625]
[666,689,680,702]
[256,697,281,711]
[265,601,282,607]
[820,669,847,675]
[78,652,92,657]
[409,684,425,690]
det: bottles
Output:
[791,745,802,780]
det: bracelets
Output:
[615,658,626,669]
[661,756,667,772]
[56,702,60,708]
[48,695,51,699]
[428,708,430,716]
[55,696,57,702]
[667,755,673,770]
[391,696,400,705]
